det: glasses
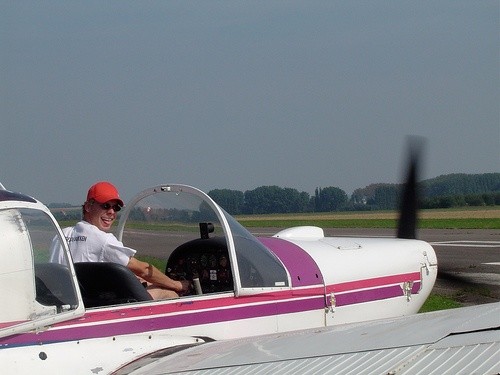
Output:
[89,201,122,212]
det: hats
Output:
[87,181,124,207]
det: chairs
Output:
[74,262,154,308]
[34,263,77,314]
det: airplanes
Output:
[0,131,500,375]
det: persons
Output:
[48,183,190,301]
